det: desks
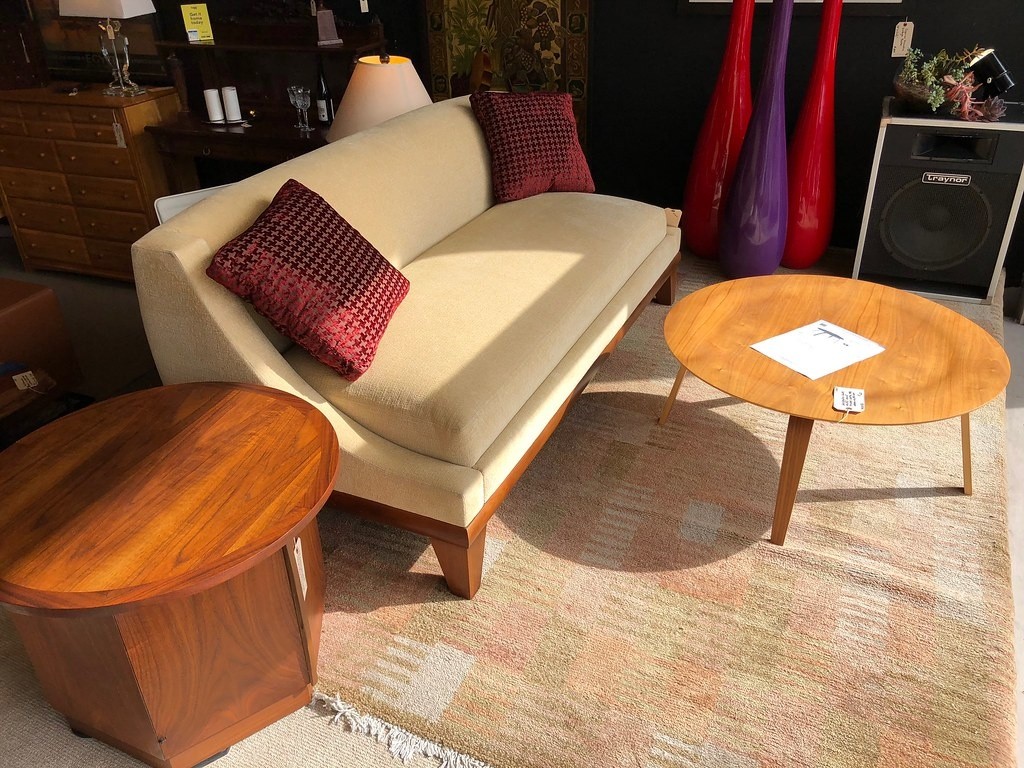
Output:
[658,275,1016,549]
[144,101,328,192]
[1,359,341,768]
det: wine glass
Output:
[287,86,307,127]
[295,88,315,131]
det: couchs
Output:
[132,94,684,599]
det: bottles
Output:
[314,54,334,127]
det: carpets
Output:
[300,261,1009,766]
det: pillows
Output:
[465,93,601,206]
[202,179,413,381]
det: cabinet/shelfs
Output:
[0,76,203,284]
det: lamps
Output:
[59,0,156,98]
[326,53,435,142]
